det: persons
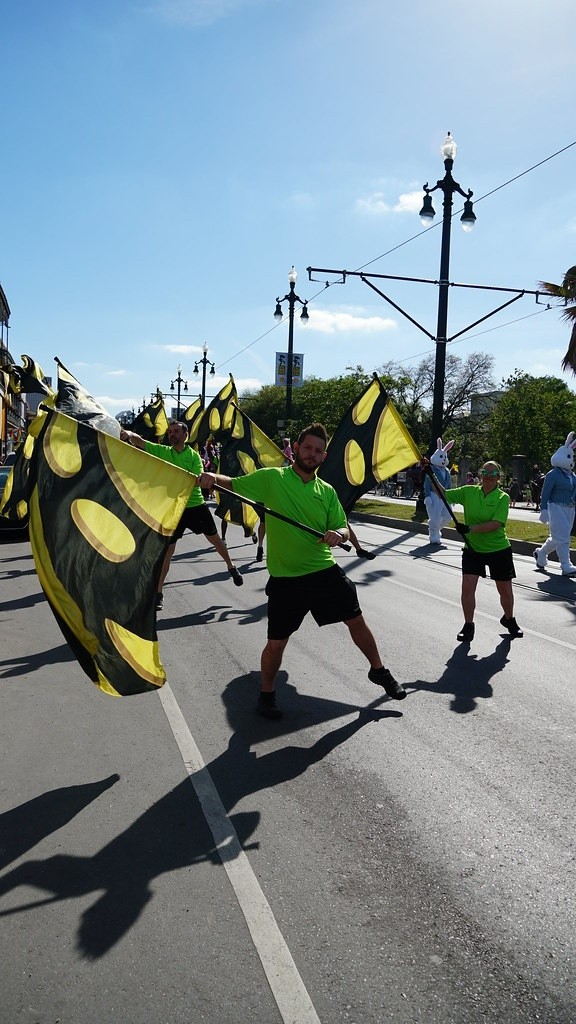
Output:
[119,422,244,588]
[281,434,547,521]
[196,422,408,722]
[426,461,524,641]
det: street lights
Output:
[193,341,216,457]
[273,264,310,438]
[411,130,478,521]
[170,363,189,423]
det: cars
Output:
[0,452,30,540]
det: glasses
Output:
[481,469,499,476]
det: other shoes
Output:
[356,548,376,560]
[562,565,576,575]
[256,690,283,722]
[457,622,475,641]
[533,551,544,569]
[222,539,227,547]
[156,592,164,610]
[256,546,264,562]
[500,614,523,637]
[252,532,258,544]
[228,565,243,586]
[367,667,406,700]
[430,541,448,549]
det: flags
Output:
[137,372,422,521]
[0,353,200,698]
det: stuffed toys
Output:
[535,430,576,580]
[423,439,456,551]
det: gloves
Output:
[455,523,470,534]
[539,509,549,523]
[425,496,432,506]
[423,462,434,476]
